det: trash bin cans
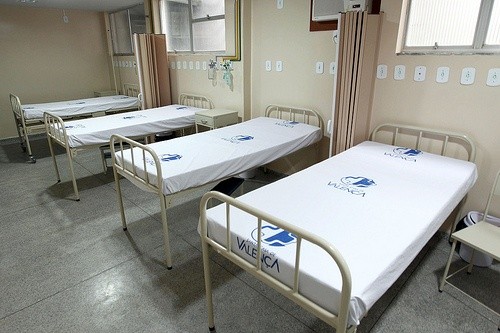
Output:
[239,168,259,179]
[459,210,500,266]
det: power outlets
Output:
[202,61,207,71]
[376,64,388,80]
[330,62,336,75]
[188,60,193,70]
[461,67,476,85]
[436,66,450,83]
[171,61,176,70]
[112,60,137,68]
[275,60,283,73]
[393,64,406,81]
[486,67,500,87]
[413,65,427,82]
[266,60,272,72]
[315,61,324,73]
[195,61,200,70]
[183,61,187,69]
[177,61,181,70]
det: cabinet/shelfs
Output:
[195,108,238,133]
[92,89,116,97]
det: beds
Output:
[42,91,213,202]
[9,83,144,164]
[108,103,325,271]
[196,121,477,333]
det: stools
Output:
[99,139,144,176]
[209,177,246,198]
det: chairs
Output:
[438,168,500,331]
